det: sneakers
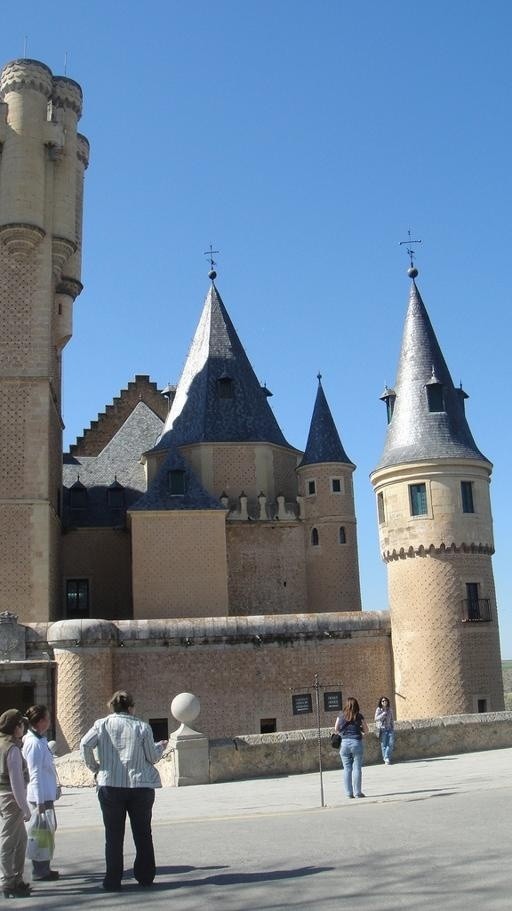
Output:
[32,871,58,881]
[346,795,355,798]
[355,793,364,797]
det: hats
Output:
[0,708,29,733]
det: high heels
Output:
[3,883,32,898]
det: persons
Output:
[22,705,60,881]
[80,690,168,893]
[374,697,395,765]
[332,697,368,799]
[0,708,32,898]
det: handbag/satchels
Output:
[24,808,56,861]
[331,734,341,748]
[376,727,381,739]
[56,785,61,800]
